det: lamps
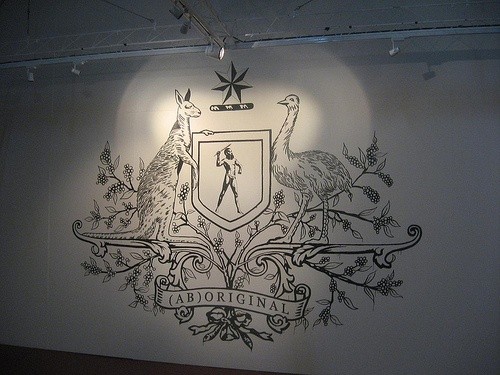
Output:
[180,15,191,34]
[205,41,225,61]
[26,69,34,82]
[72,64,81,76]
[389,39,400,56]
